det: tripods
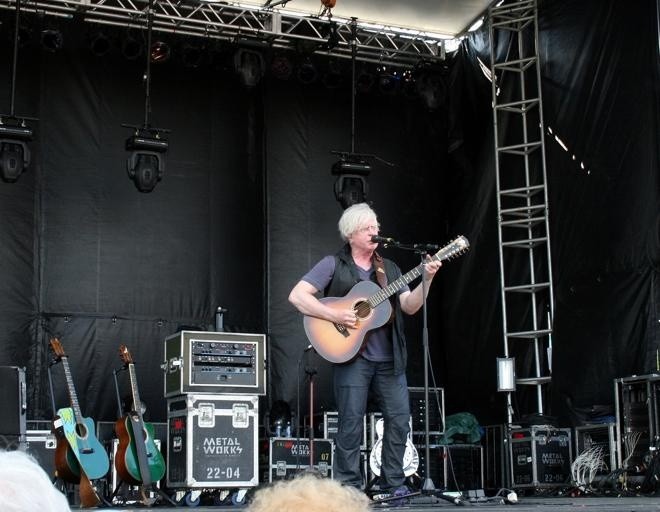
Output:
[368,280,472,506]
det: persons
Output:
[0,448,74,512]
[286,200,443,492]
[243,471,374,512]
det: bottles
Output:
[285,422,292,437]
[275,421,283,437]
[215,305,224,332]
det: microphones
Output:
[369,233,394,246]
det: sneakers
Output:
[388,486,411,505]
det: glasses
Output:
[352,222,380,233]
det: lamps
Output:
[330,17,394,209]
[1,1,39,184]
[120,9,174,194]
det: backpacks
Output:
[440,413,484,445]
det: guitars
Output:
[49,338,110,481]
[116,345,165,485]
[369,418,419,478]
[304,234,469,366]
